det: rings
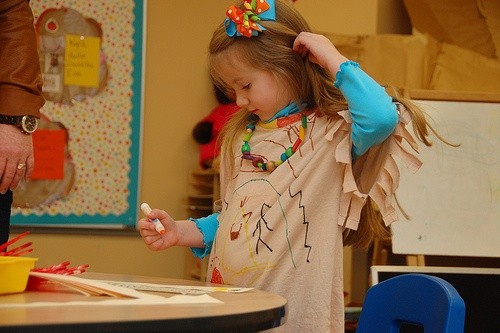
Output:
[17,163,26,169]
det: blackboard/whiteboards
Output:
[385,87,500,257]
[371,266,500,333]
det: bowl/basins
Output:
[0,255,39,294]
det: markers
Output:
[140,201,166,235]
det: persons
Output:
[138,0,462,333]
[0,0,46,252]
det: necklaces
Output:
[241,113,307,172]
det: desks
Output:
[0,272,287,333]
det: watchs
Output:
[0,114,38,135]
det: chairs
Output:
[357,273,466,333]
